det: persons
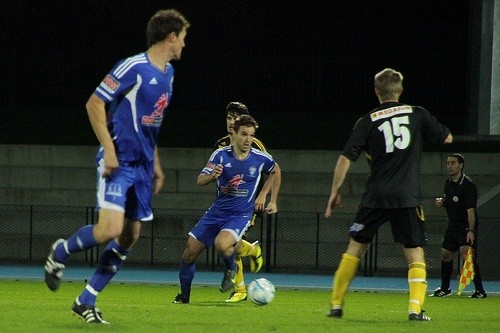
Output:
[215,102,281,303]
[44,9,189,324]
[429,153,487,299]
[173,114,277,303]
[324,68,454,321]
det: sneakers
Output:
[326,309,343,318]
[471,290,487,298]
[42,237,67,291]
[171,293,190,304]
[429,286,452,298]
[250,240,263,272]
[224,290,248,303]
[219,263,240,292]
[70,296,110,325]
[408,310,434,322]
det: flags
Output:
[457,248,474,295]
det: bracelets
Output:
[470,230,475,233]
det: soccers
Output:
[248,278,276,305]
